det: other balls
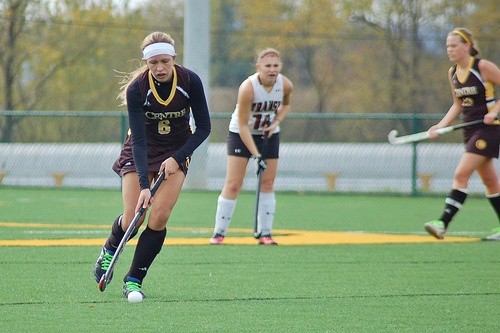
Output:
[128,290,143,303]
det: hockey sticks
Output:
[99,168,165,293]
[387,116,498,146]
[254,120,271,240]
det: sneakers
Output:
[210,232,225,245]
[423,219,446,239]
[482,231,500,241]
[124,274,146,301]
[259,233,278,246]
[94,246,118,283]
